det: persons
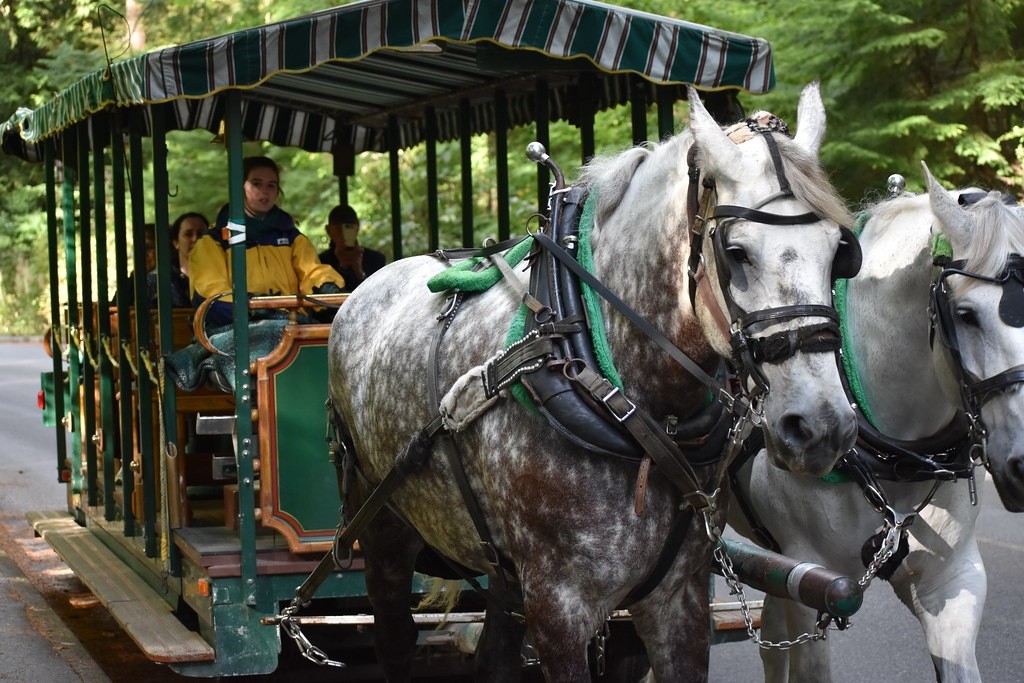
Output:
[147,212,211,308]
[318,203,385,294]
[188,156,347,477]
[111,223,157,307]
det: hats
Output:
[328,204,360,224]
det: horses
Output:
[328,78,1024,683]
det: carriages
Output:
[3,1,1023,683]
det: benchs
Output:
[66,303,235,530]
